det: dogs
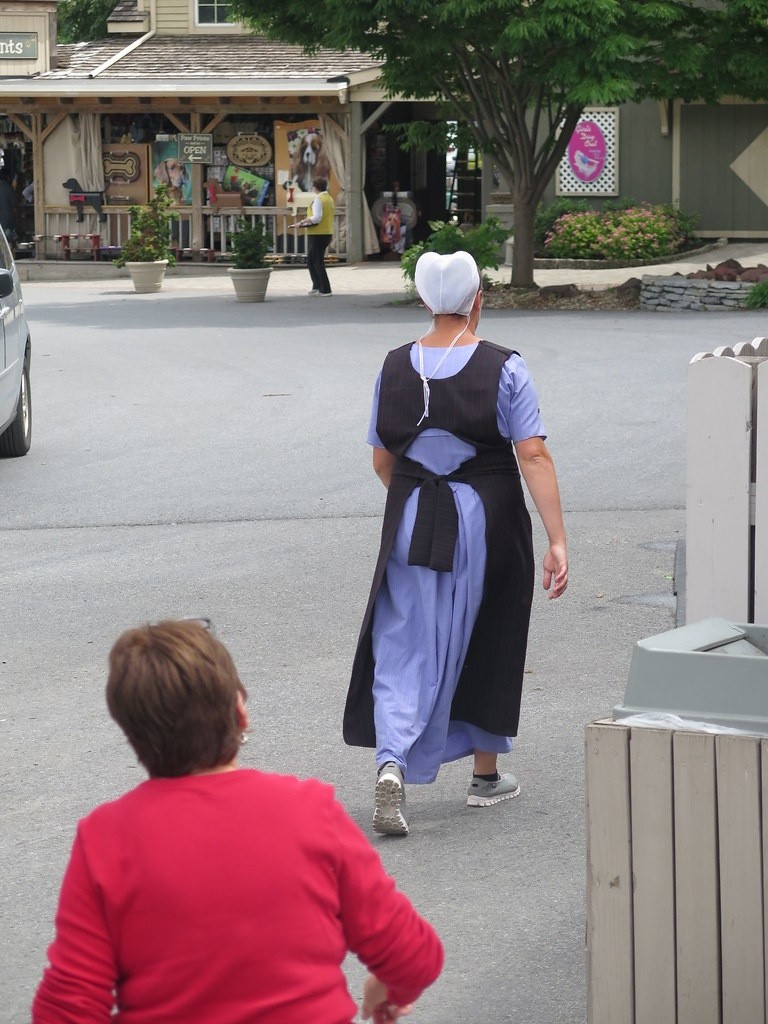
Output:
[154,157,189,206]
[62,177,111,223]
[294,132,332,192]
[383,211,400,242]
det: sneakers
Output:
[466,773,520,807]
[372,762,409,834]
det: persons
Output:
[31,619,444,1023]
[0,166,34,232]
[343,248,568,834]
[295,176,335,297]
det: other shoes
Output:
[308,289,319,295]
[313,293,332,297]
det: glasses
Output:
[174,615,215,640]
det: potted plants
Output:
[225,214,273,303]
[112,181,179,294]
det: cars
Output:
[0,224,32,457]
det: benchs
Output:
[177,248,214,263]
[99,246,179,262]
[59,234,100,261]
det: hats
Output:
[413,251,479,317]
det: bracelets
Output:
[301,220,303,224]
